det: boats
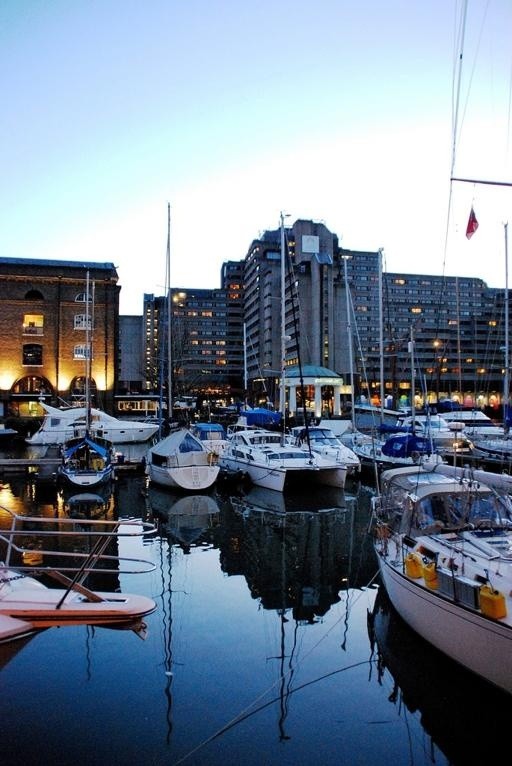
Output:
[0,505,158,672]
[147,486,220,555]
[365,585,512,766]
[233,491,359,519]
[61,488,113,550]
[372,465,512,693]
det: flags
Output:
[465,208,478,239]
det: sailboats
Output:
[55,271,119,492]
[142,201,222,494]
[0,202,512,489]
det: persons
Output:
[76,443,104,472]
